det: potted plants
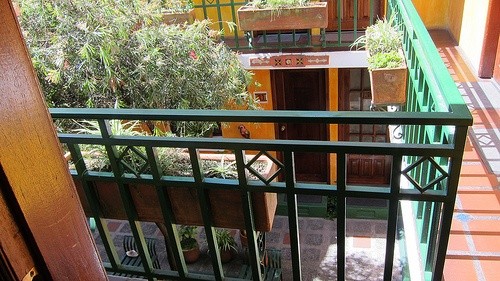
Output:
[237,1,329,30]
[177,224,201,264]
[62,99,279,232]
[145,0,194,27]
[349,5,408,105]
[206,228,238,264]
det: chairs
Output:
[239,248,283,280]
[113,236,161,279]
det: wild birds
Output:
[238,124,250,139]
